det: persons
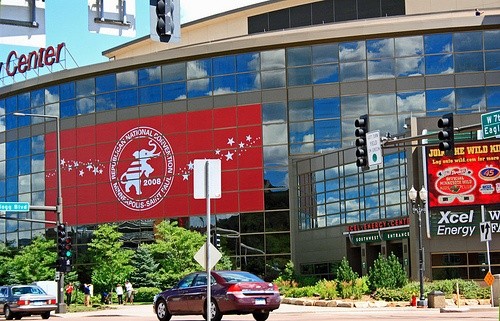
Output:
[124,280,134,306]
[101,292,111,304]
[84,283,91,307]
[116,284,124,305]
[65,282,75,307]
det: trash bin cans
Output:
[491,274,500,308]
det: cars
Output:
[153,271,281,321]
[0,285,58,320]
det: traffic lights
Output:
[56,222,73,272]
[355,113,370,173]
[437,112,455,158]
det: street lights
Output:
[12,112,67,315]
[409,185,428,308]
